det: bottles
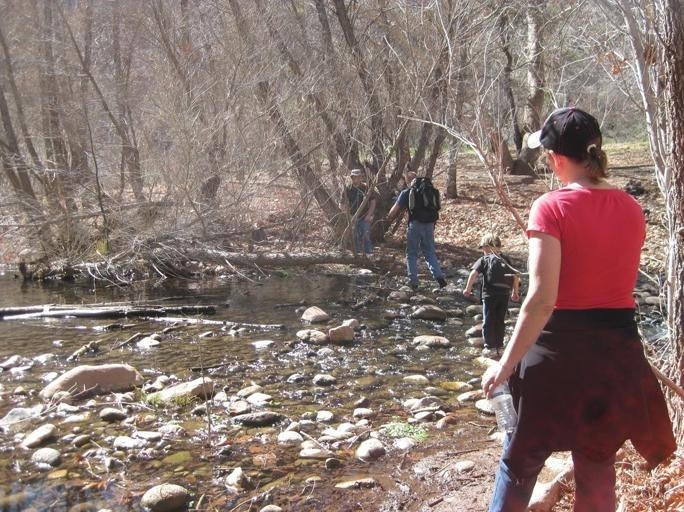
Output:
[482,360,518,434]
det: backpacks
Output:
[486,253,513,289]
[409,176,439,221]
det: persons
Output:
[462,233,521,357]
[386,171,447,291]
[481,107,646,512]
[338,169,378,253]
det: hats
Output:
[478,233,502,249]
[350,169,361,177]
[526,107,602,150]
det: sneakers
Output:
[406,278,447,289]
[481,347,504,357]
